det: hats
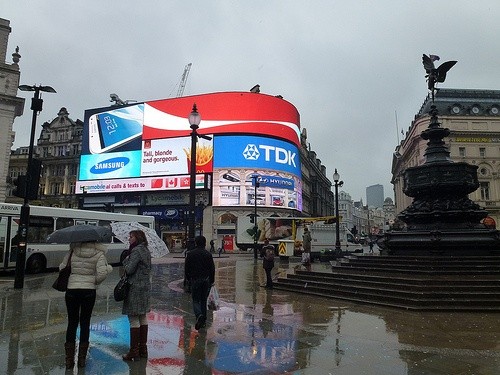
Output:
[264,238,269,244]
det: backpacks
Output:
[264,249,274,261]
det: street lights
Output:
[187,101,201,251]
[12,83,57,290]
[330,168,344,255]
[251,172,261,260]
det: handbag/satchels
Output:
[207,286,220,311]
[52,251,73,291]
[301,251,310,263]
[114,273,130,302]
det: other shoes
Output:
[195,315,204,330]
[265,287,273,290]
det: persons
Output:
[184,236,215,330]
[119,230,152,360]
[263,249,274,290]
[369,241,374,252]
[210,239,215,252]
[303,227,312,264]
[58,241,113,368]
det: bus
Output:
[0,202,156,275]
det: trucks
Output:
[235,215,364,262]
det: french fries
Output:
[183,142,213,164]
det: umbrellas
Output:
[46,224,117,245]
[260,245,275,257]
[110,221,169,259]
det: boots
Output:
[78,341,89,366]
[121,327,141,361]
[140,324,148,357]
[65,342,76,368]
